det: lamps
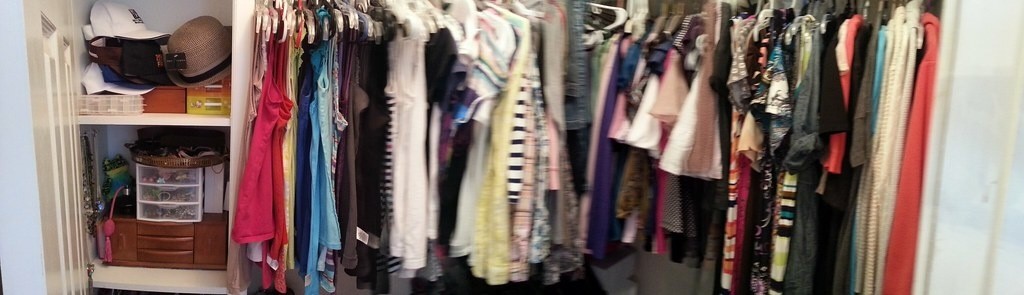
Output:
[108,182,134,220]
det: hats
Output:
[81,1,177,95]
[160,16,232,88]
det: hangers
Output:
[251,0,943,71]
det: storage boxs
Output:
[139,74,231,115]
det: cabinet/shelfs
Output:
[102,211,228,271]
[135,162,204,222]
[67,0,256,295]
